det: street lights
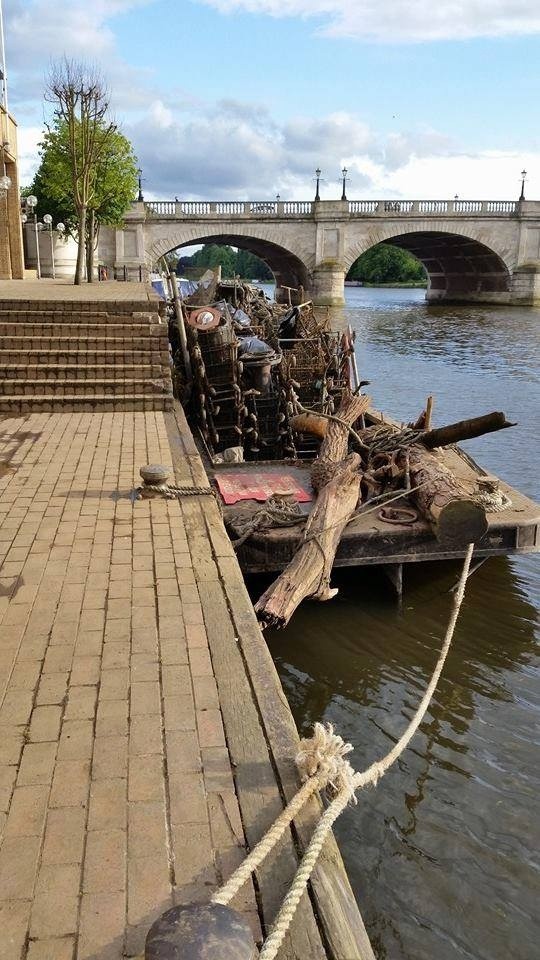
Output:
[339,166,350,200]
[518,169,528,200]
[135,166,146,202]
[312,168,324,201]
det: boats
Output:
[152,277,197,299]
[251,280,264,284]
[344,279,363,287]
[170,267,540,574]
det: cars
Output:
[250,205,275,214]
